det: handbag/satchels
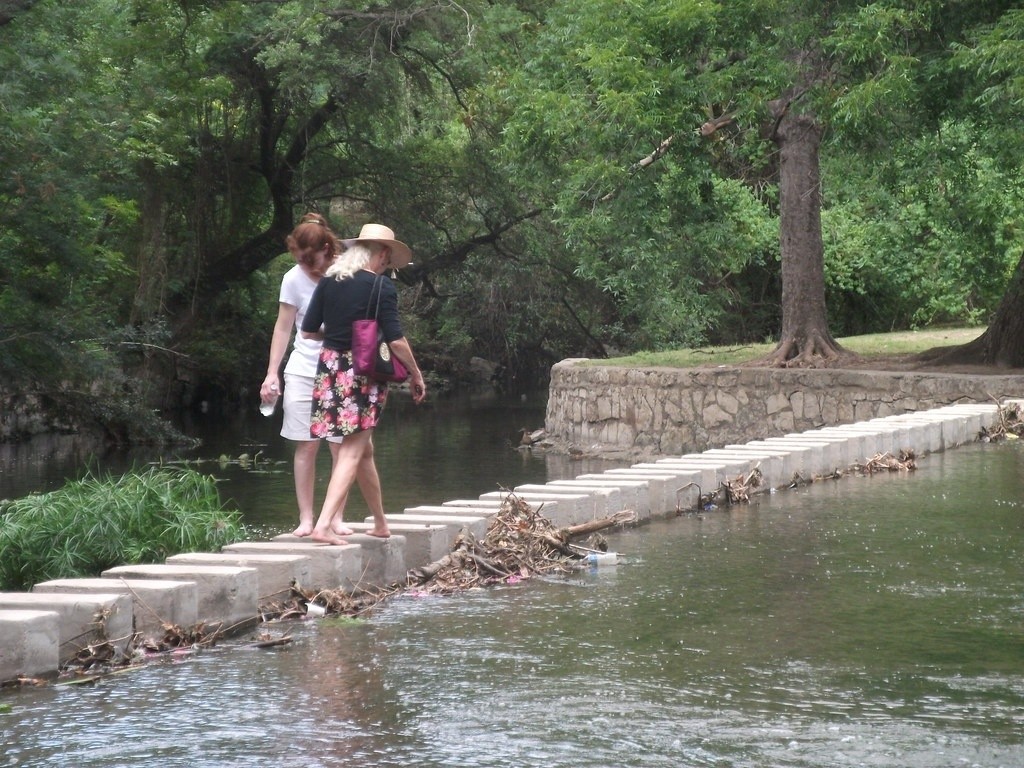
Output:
[353,320,408,382]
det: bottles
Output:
[260,385,278,416]
[580,553,616,565]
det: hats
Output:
[338,223,411,269]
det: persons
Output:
[259,213,353,537]
[300,224,425,545]
